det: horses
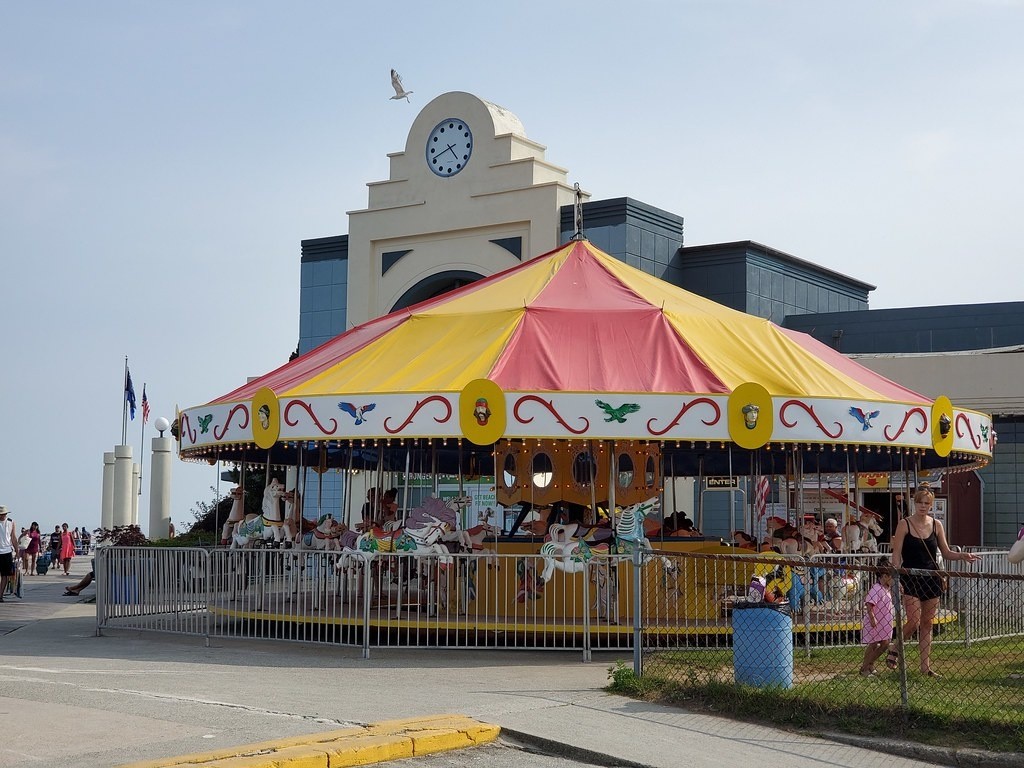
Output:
[549,512,621,543]
[828,563,869,616]
[734,516,833,570]
[281,488,317,541]
[221,486,258,546]
[226,478,292,557]
[538,496,683,588]
[287,513,343,571]
[332,496,456,574]
[841,513,884,554]
[413,495,473,579]
[459,521,501,572]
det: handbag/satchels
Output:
[16,533,32,550]
[941,576,948,591]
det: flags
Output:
[125,367,136,421]
[142,389,150,424]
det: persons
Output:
[891,481,983,678]
[171,419,178,437]
[819,518,846,602]
[992,430,998,445]
[62,571,96,596]
[18,522,91,576]
[1009,526,1024,679]
[169,517,174,539]
[474,398,491,426]
[939,413,951,438]
[357,487,398,600]
[0,505,19,603]
[664,511,693,532]
[859,556,895,678]
[258,405,270,430]
[560,508,569,525]
[742,404,759,429]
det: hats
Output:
[0,505,11,514]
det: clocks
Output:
[424,118,473,177]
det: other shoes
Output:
[869,662,880,676]
[51,566,56,569]
[0,597,4,602]
[57,565,60,569]
[65,586,80,595]
[31,572,35,576]
[63,571,70,576]
[23,572,28,576]
[859,667,875,678]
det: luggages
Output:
[36,551,48,575]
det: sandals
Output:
[886,642,898,670]
[921,669,942,679]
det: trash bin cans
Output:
[731,601,793,689]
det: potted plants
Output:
[90,524,156,604]
